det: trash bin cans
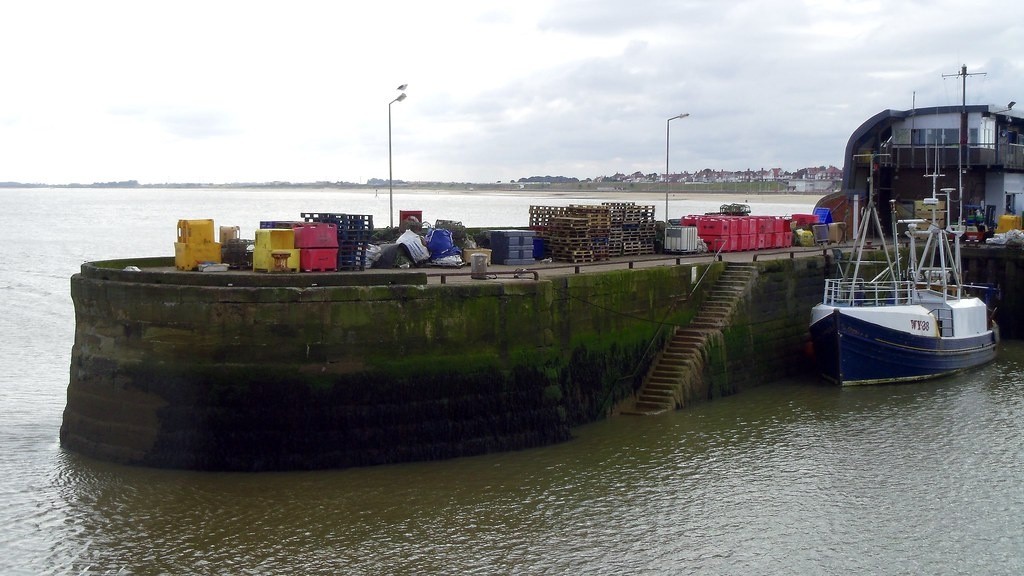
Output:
[470,253,489,279]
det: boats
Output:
[804,170,1000,389]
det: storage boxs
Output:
[811,207,833,244]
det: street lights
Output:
[389,93,407,229]
[665,113,690,223]
